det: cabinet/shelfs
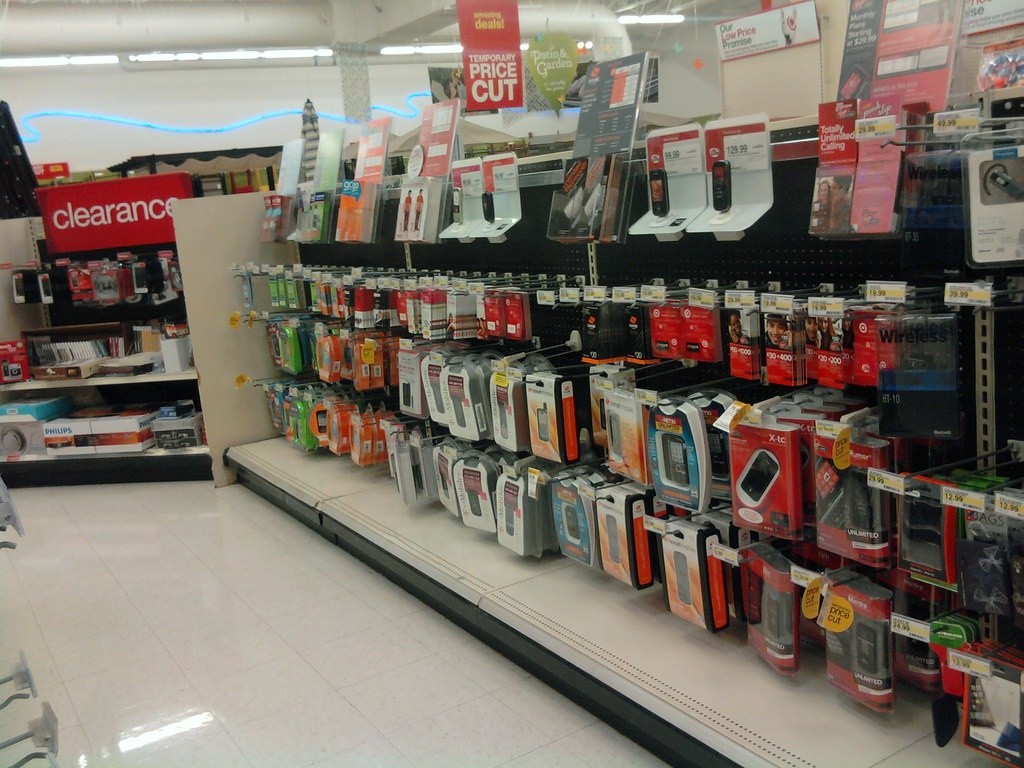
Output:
[0,366,220,486]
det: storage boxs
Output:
[0,398,206,457]
[31,357,109,380]
[158,336,192,373]
[97,363,154,376]
